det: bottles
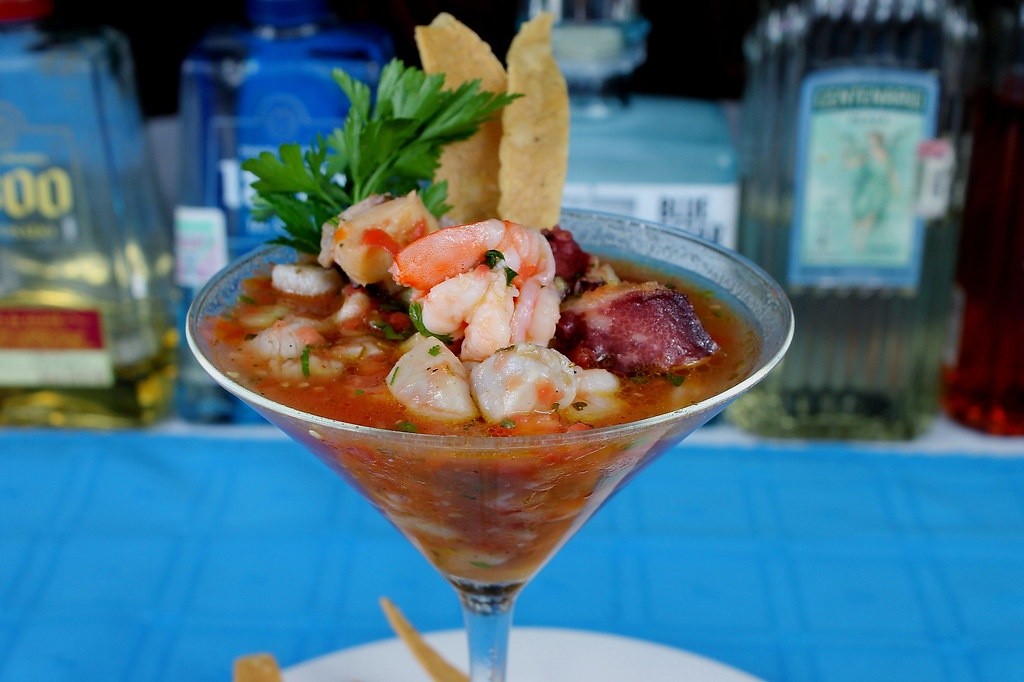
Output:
[525,0,739,248]
[170,59,230,420]
[235,0,388,258]
[724,0,977,438]
[0,0,177,432]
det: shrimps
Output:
[252,186,621,450]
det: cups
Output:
[941,0,1024,436]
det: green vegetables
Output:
[240,58,525,431]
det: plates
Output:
[282,626,764,682]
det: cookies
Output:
[499,12,570,229]
[412,11,505,222]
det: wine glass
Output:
[186,208,795,682]
